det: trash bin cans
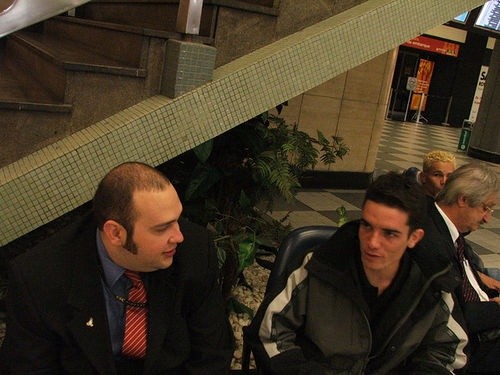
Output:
[455,119,475,155]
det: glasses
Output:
[483,201,497,216]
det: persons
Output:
[400,149,500,375]
[246,172,470,375]
[0,162,235,375]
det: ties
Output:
[120,270,148,358]
[457,234,481,303]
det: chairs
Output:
[242,225,339,375]
